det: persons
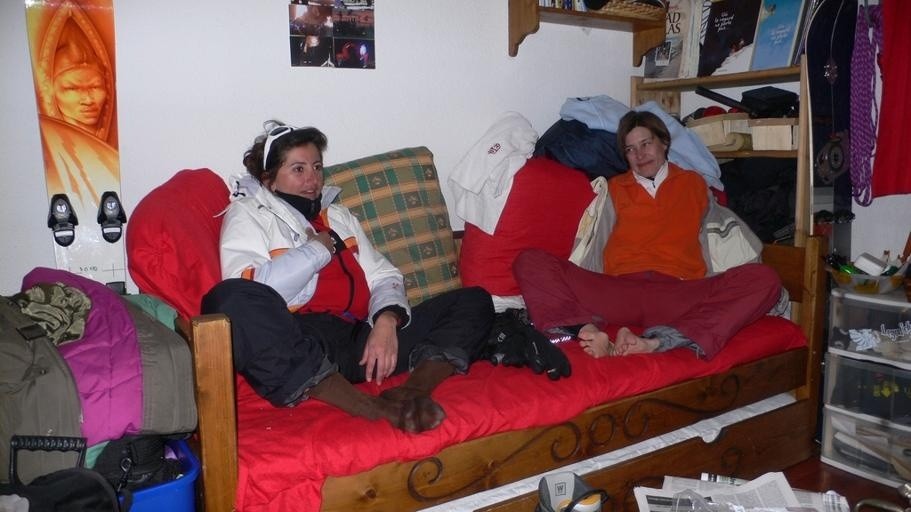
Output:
[51,41,107,137]
[512,105,784,363]
[197,121,494,435]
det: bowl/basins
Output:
[825,261,906,295]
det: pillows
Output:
[319,146,465,309]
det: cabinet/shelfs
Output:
[509,0,667,67]
[820,287,911,489]
[630,53,810,325]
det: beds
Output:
[139,234,829,512]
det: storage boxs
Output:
[749,117,799,152]
[115,436,202,512]
[686,111,748,148]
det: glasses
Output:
[263,123,298,166]
